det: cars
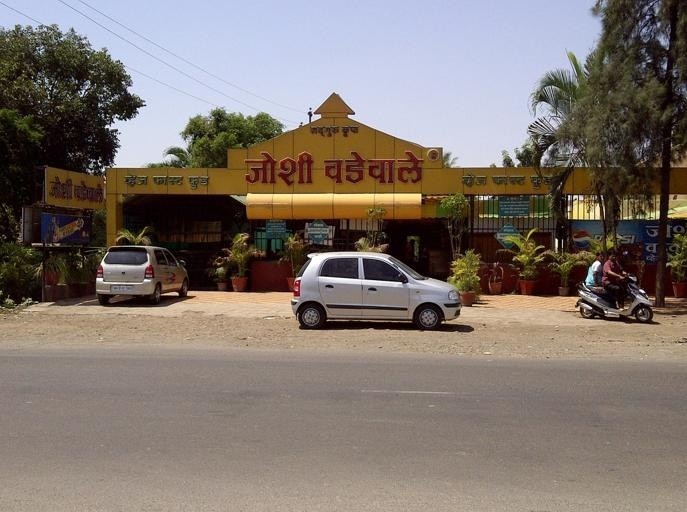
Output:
[291,252,461,329]
[96,245,189,304]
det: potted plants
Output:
[221,233,266,292]
[0,243,106,302]
[278,230,305,292]
[216,267,228,291]
[446,227,687,307]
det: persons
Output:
[601,246,632,313]
[584,251,606,290]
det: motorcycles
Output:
[575,273,653,323]
